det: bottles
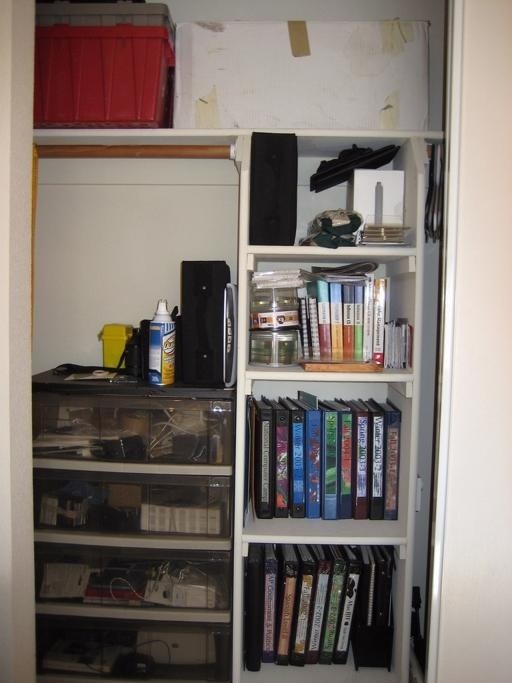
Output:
[148,300,175,386]
[250,286,299,330]
[252,332,296,366]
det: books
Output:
[245,543,396,671]
[245,391,400,518]
[297,260,410,369]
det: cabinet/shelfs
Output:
[31,130,237,683]
[233,129,446,683]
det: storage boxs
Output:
[29,0,174,129]
[174,20,430,131]
